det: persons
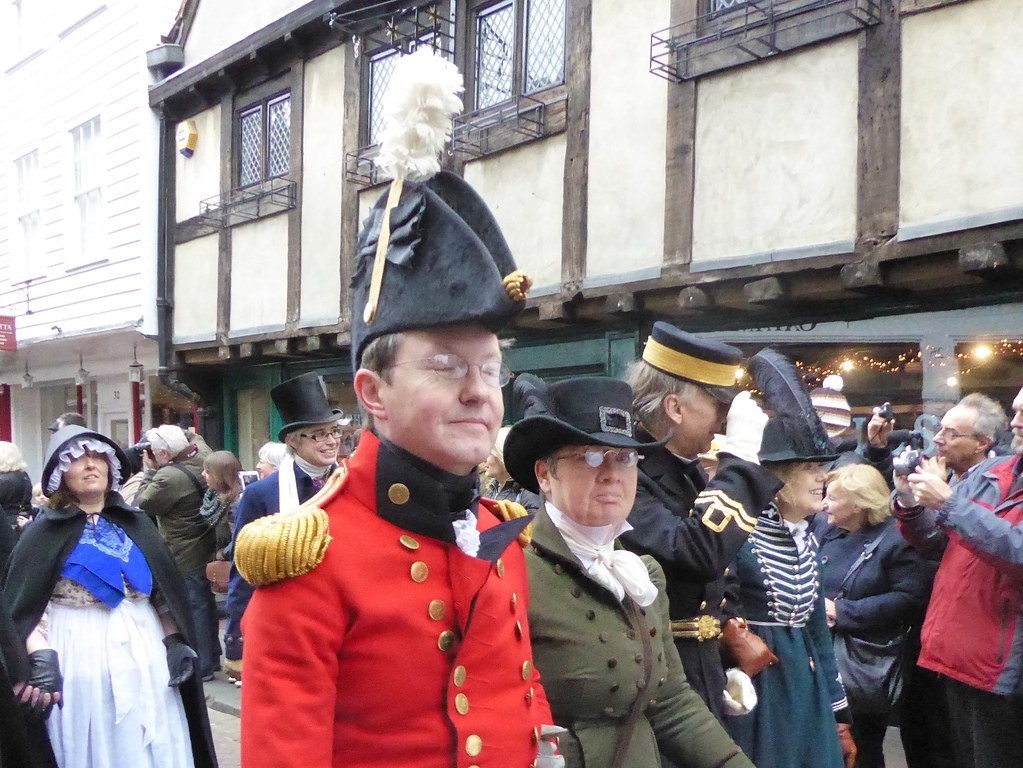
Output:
[0,424,217,768]
[234,42,569,768]
[0,319,1023,768]
[821,461,940,767]
[859,392,1023,767]
[721,344,858,768]
[132,424,223,682]
[618,320,788,728]
[502,371,756,767]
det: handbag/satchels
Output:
[833,630,909,726]
[206,560,232,594]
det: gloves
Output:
[716,392,770,463]
[724,618,778,680]
[17,649,63,714]
[164,633,198,686]
[721,670,758,715]
[836,722,857,767]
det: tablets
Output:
[239,470,260,491]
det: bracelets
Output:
[724,624,749,640]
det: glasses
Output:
[553,446,646,468]
[300,429,343,442]
[376,354,514,387]
[934,423,987,440]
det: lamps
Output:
[128,341,145,382]
[74,352,91,385]
[21,361,34,389]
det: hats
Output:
[504,376,672,495]
[271,371,344,444]
[41,424,130,498]
[349,45,531,381]
[746,344,840,465]
[643,321,742,404]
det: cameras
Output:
[878,402,894,422]
[133,442,152,455]
[893,449,921,476]
